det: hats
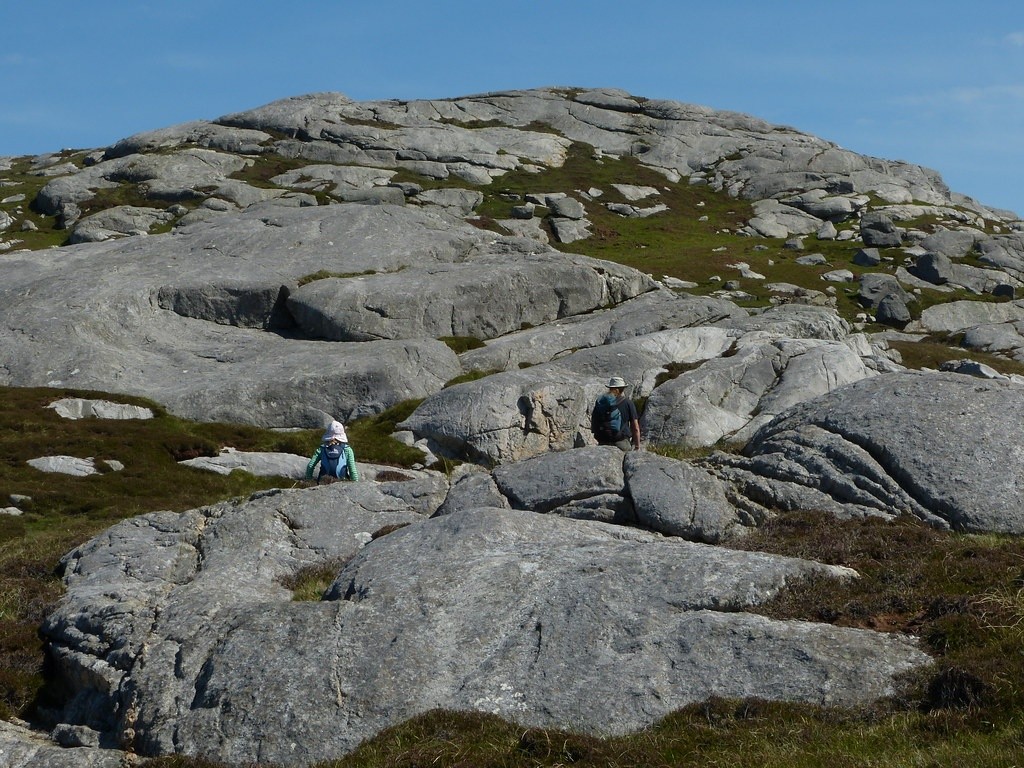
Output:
[322,421,348,443]
[605,377,628,387]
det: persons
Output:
[592,376,641,452]
[305,421,358,487]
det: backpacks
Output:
[316,443,349,485]
[592,394,629,443]
